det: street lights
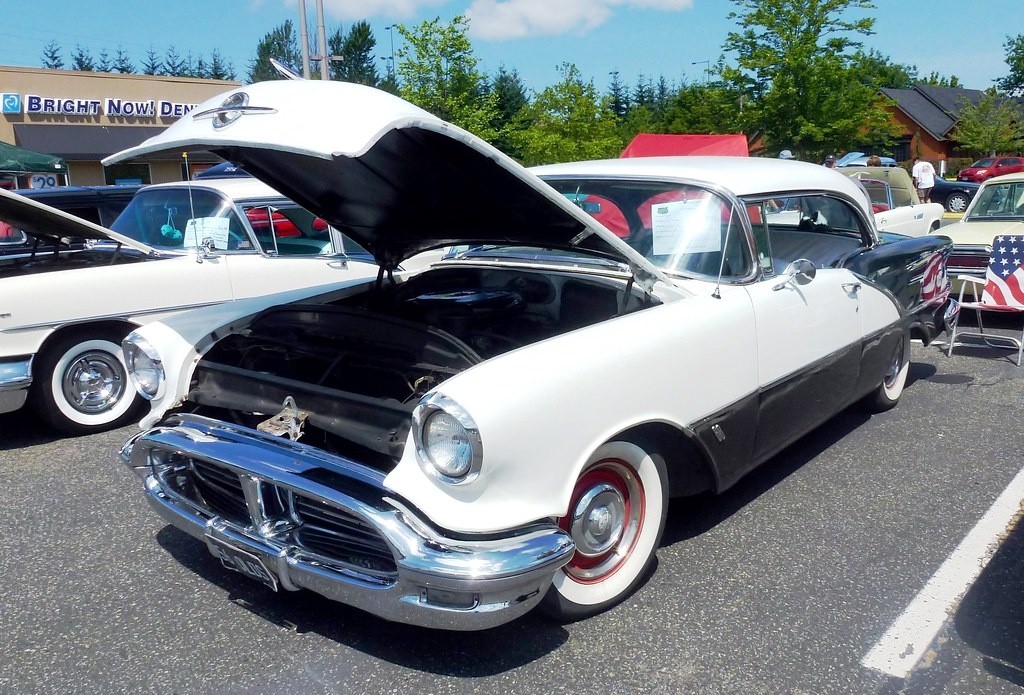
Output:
[385,24,398,83]
[692,60,709,84]
[380,56,393,78]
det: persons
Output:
[779,149,795,160]
[824,155,837,168]
[866,156,882,167]
[912,157,937,204]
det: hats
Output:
[779,150,795,159]
[825,155,837,164]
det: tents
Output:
[0,140,68,190]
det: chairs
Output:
[944,234,1024,366]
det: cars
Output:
[765,152,946,253]
[100,77,959,631]
[935,175,1001,213]
[930,173,1024,298]
[957,156,1024,182]
[1,191,397,432]
[92,178,456,274]
[0,187,245,259]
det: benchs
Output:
[259,236,331,254]
[725,226,857,276]
